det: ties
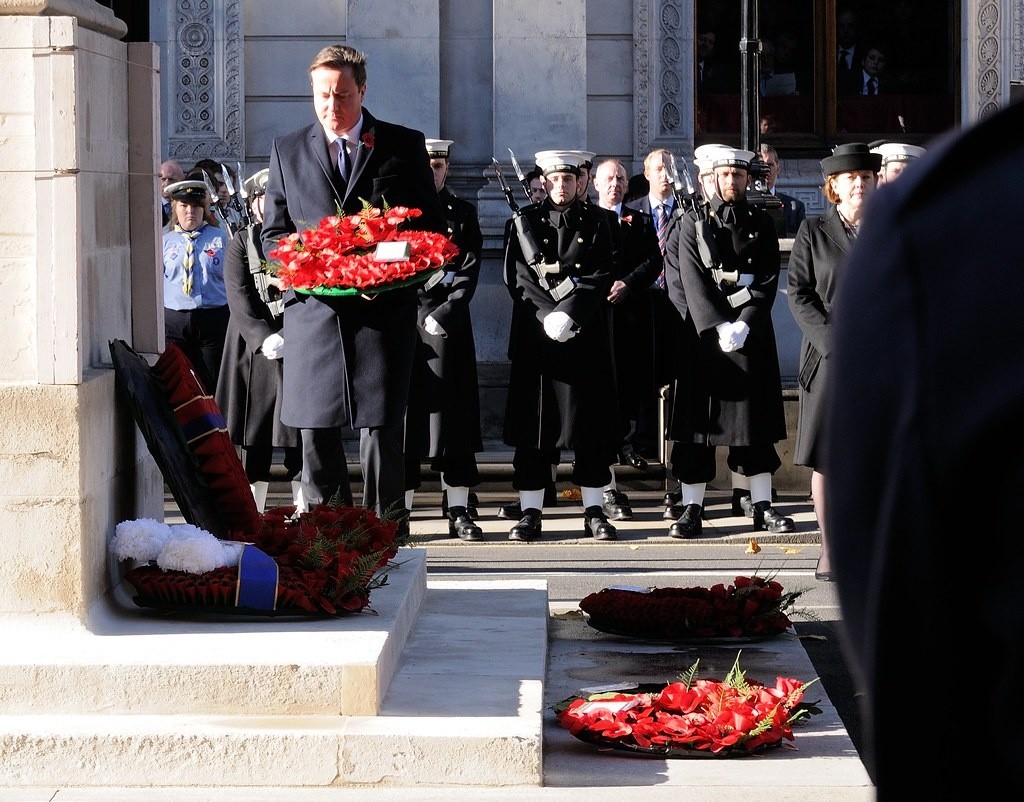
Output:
[867,77,876,96]
[838,50,849,77]
[655,203,668,289]
[172,221,208,298]
[334,138,352,200]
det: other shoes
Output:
[815,556,834,583]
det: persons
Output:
[162,117,927,548]
[659,148,796,539]
[261,44,438,548]
[502,148,627,540]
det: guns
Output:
[491,148,578,303]
[670,153,755,309]
[202,162,285,323]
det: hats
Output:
[244,169,270,196]
[163,180,208,200]
[820,139,927,176]
[425,137,454,160]
[530,142,756,175]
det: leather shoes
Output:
[373,490,485,542]
[499,477,634,542]
[661,480,796,536]
[620,452,651,471]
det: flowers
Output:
[356,126,376,149]
[621,215,632,226]
[154,344,259,532]
[204,249,216,256]
[107,484,418,616]
[579,553,838,638]
[555,647,823,757]
[258,199,461,291]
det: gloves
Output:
[544,312,577,342]
[714,320,750,352]
[423,315,447,338]
[259,335,286,359]
